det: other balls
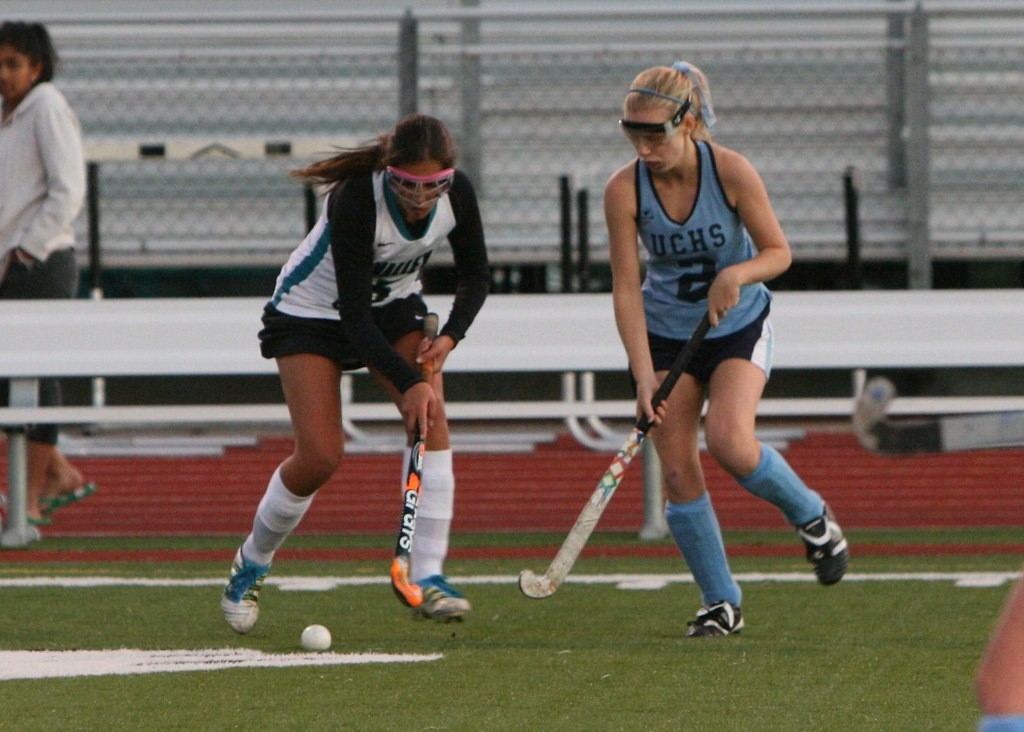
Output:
[297,624,332,651]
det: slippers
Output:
[34,479,97,516]
[0,502,51,528]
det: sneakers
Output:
[687,603,743,636]
[797,501,848,586]
[221,542,269,635]
[408,576,469,625]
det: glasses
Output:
[379,156,457,205]
[618,93,694,146]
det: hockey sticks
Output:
[518,308,712,600]
[391,312,441,608]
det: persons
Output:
[603,61,850,636]
[219,115,491,636]
[1,19,89,524]
